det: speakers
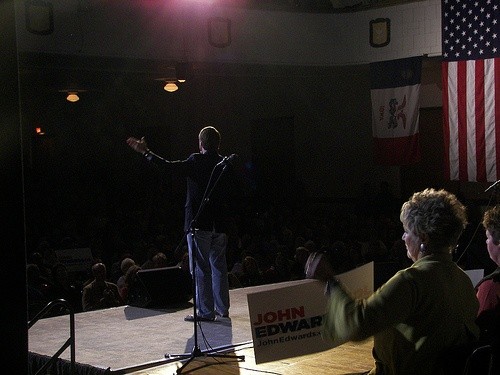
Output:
[133,266,193,309]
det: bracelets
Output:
[143,150,150,156]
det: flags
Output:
[441,0,500,182]
[368,57,424,165]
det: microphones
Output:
[220,154,239,166]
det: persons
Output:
[127,127,233,325]
[474,205,500,375]
[23,213,409,320]
[304,188,480,375]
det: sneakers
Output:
[184,315,215,322]
[214,310,229,318]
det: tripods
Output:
[165,162,245,375]
